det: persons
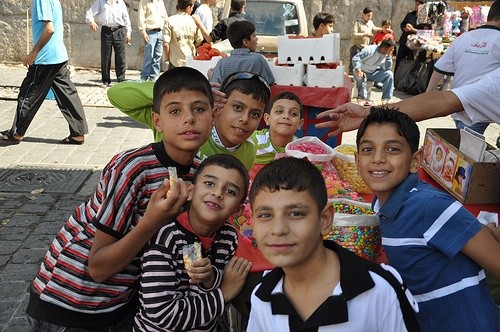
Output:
[441,157,454,181]
[107,71,272,173]
[452,166,466,195]
[312,0,500,137]
[131,154,253,332]
[0,0,89,144]
[354,105,500,332]
[424,144,432,165]
[24,67,218,332]
[246,157,424,332]
[85,0,248,87]
[432,147,443,174]
[208,21,275,90]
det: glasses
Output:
[222,71,271,98]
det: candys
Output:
[231,143,381,263]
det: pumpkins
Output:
[194,42,220,60]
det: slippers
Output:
[0,131,19,145]
[59,136,83,145]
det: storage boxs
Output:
[277,31,340,64]
[268,57,305,86]
[186,52,230,81]
[420,127,500,204]
[306,65,345,89]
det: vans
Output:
[216,0,308,56]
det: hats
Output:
[382,38,395,46]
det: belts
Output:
[108,27,117,30]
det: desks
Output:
[259,74,351,147]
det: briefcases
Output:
[393,53,431,94]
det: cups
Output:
[183,244,202,269]
